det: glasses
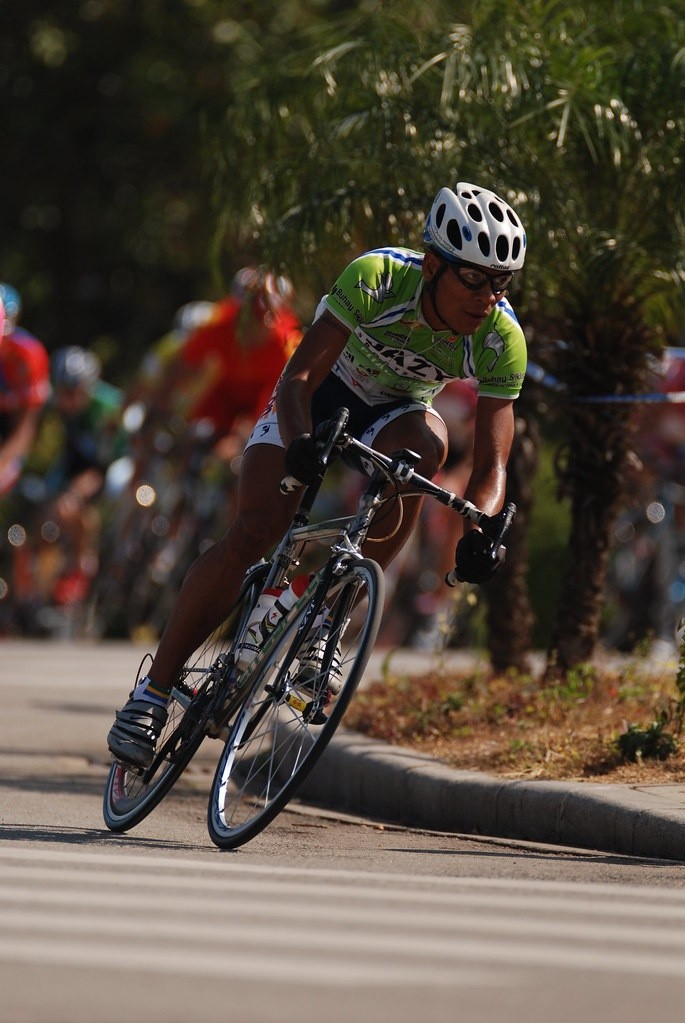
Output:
[440,255,513,294]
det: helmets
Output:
[422,181,525,273]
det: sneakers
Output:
[296,604,352,696]
[107,675,176,766]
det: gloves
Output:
[457,532,507,577]
[283,438,324,485]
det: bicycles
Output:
[100,405,521,851]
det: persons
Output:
[106,182,527,765]
[0,266,685,656]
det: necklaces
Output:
[378,314,452,365]
